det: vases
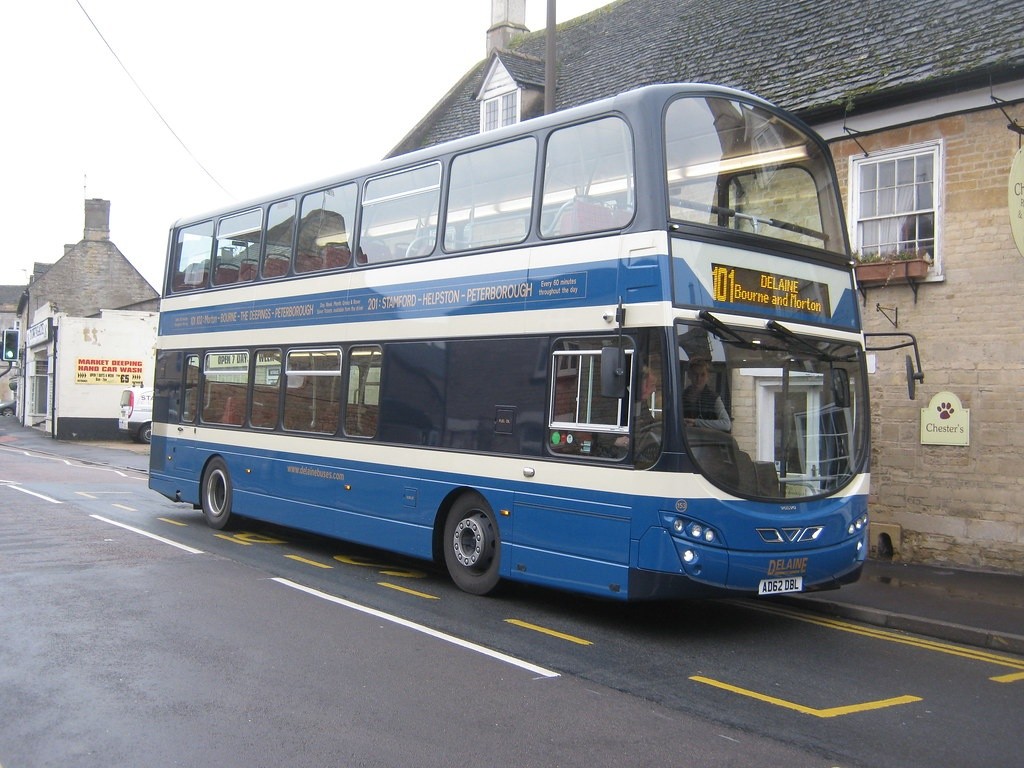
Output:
[855,260,930,283]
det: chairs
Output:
[172,203,631,292]
[182,376,388,436]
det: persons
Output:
[683,356,731,431]
[594,365,657,460]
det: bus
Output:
[148,86,923,622]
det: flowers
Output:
[852,249,927,264]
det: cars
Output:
[0,400,16,418]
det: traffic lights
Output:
[2,329,19,362]
